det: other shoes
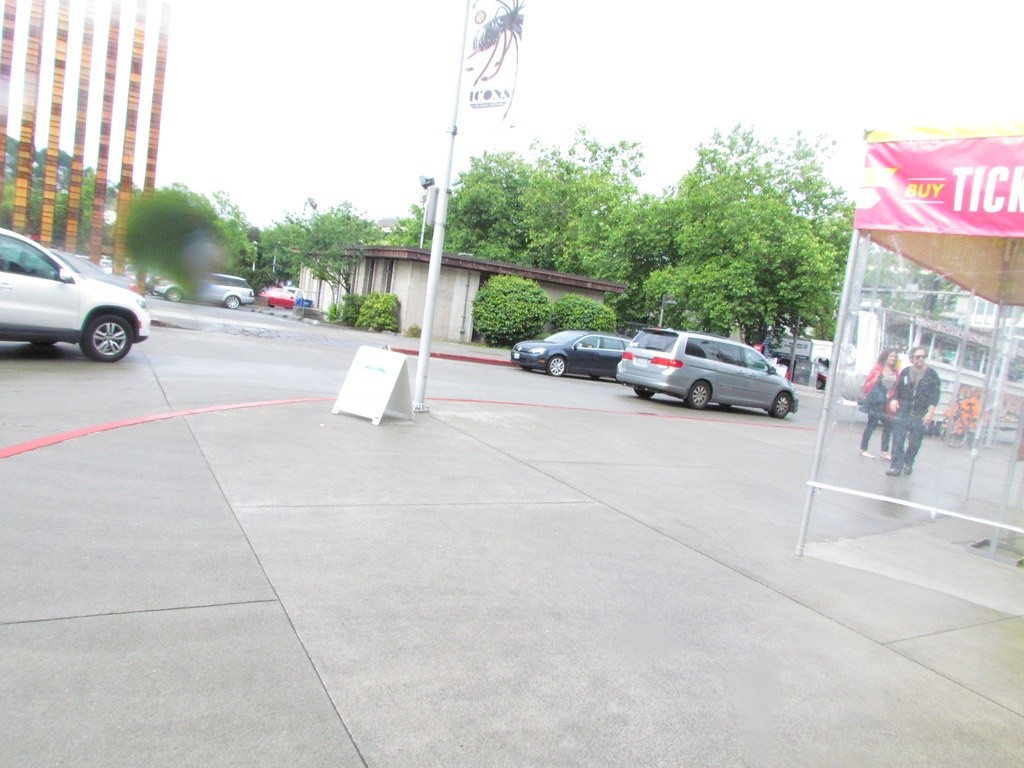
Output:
[860,451,876,458]
[881,452,891,460]
[886,468,902,476]
[904,463,913,475]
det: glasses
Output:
[912,355,926,360]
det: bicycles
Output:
[940,393,986,451]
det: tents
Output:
[796,121,1024,632]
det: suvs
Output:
[0,227,151,362]
[153,273,255,310]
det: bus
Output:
[834,300,1024,443]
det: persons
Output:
[886,347,940,476]
[859,349,899,461]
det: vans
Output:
[616,327,798,419]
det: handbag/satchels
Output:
[864,377,888,417]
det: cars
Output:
[777,358,829,389]
[283,286,299,295]
[258,289,296,310]
[0,244,139,345]
[510,330,633,386]
